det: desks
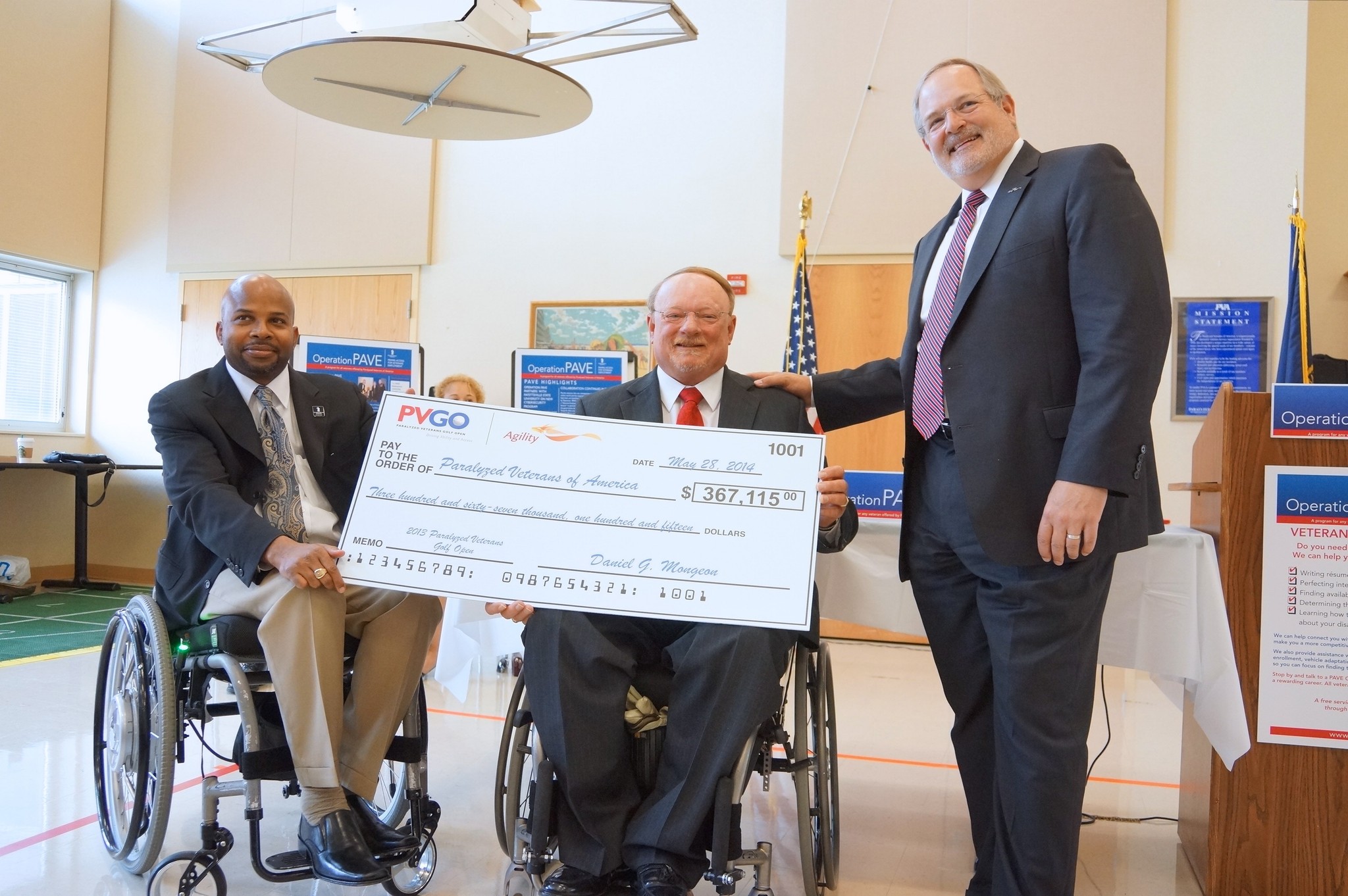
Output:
[433,519,1251,772]
[0,463,163,591]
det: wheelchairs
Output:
[91,592,446,896]
[492,584,842,896]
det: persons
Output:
[421,376,492,678]
[748,58,1171,896]
[147,272,442,887]
[358,378,385,399]
[485,265,859,896]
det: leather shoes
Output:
[344,794,421,856]
[628,863,695,896]
[296,809,391,885]
[538,864,619,896]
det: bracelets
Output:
[819,519,838,530]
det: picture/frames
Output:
[530,301,657,381]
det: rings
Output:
[840,496,849,507]
[314,568,327,579]
[512,618,517,623]
[1066,534,1081,539]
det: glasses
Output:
[652,309,732,324]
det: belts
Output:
[933,423,956,441]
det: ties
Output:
[674,387,707,427]
[252,385,309,544]
[911,188,986,440]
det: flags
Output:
[1276,213,1316,384]
[782,233,818,377]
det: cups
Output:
[17,437,35,463]
